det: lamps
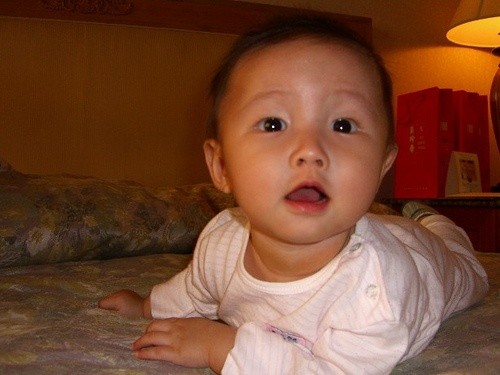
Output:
[446,0,500,192]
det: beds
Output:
[0,169,500,375]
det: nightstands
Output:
[376,194,499,256]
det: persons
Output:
[99,15,490,375]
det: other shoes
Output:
[401,200,440,221]
